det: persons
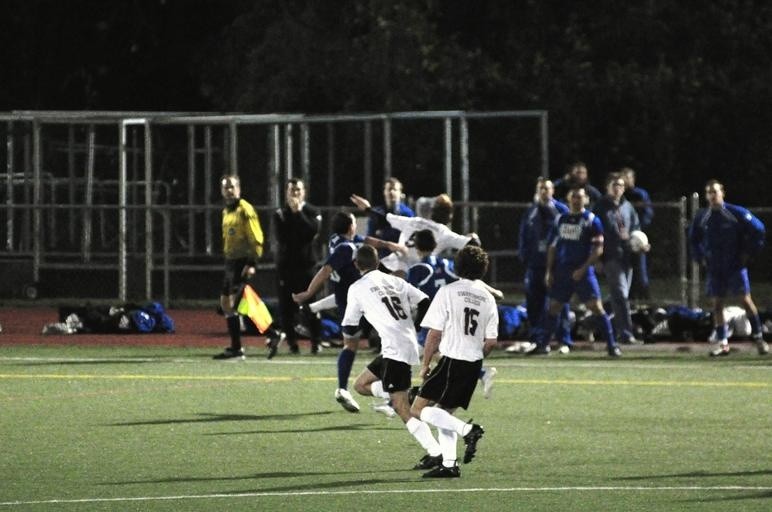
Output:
[211,173,286,363]
[408,245,500,478]
[341,245,445,470]
[291,211,408,412]
[293,163,490,354]
[498,162,771,366]
[369,229,504,419]
[273,178,324,355]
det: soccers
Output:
[630,230,648,251]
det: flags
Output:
[236,284,274,336]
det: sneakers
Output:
[499,315,672,359]
[412,450,443,473]
[708,340,733,358]
[373,400,398,420]
[481,364,499,399]
[263,329,380,362]
[421,465,465,479]
[205,343,249,362]
[753,338,770,354]
[461,422,485,463]
[332,387,360,417]
